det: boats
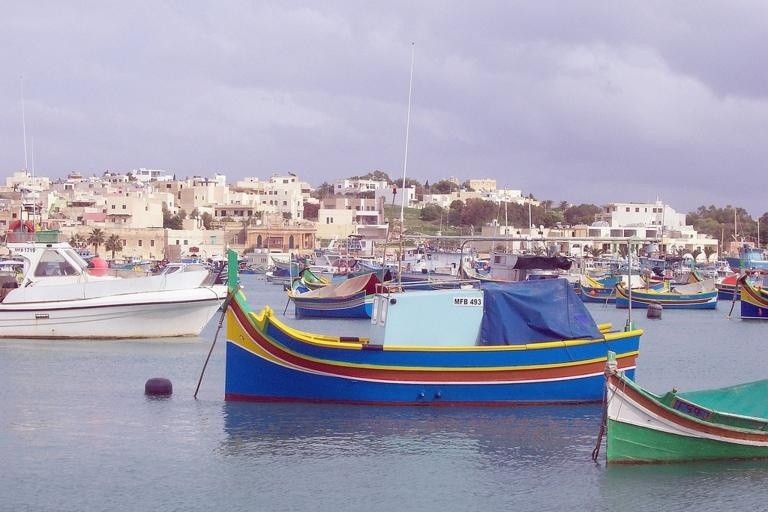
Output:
[285,271,391,319]
[615,276,719,310]
[225,41,644,407]
[728,272,768,320]
[0,75,768,302]
[0,262,230,340]
[605,350,768,465]
